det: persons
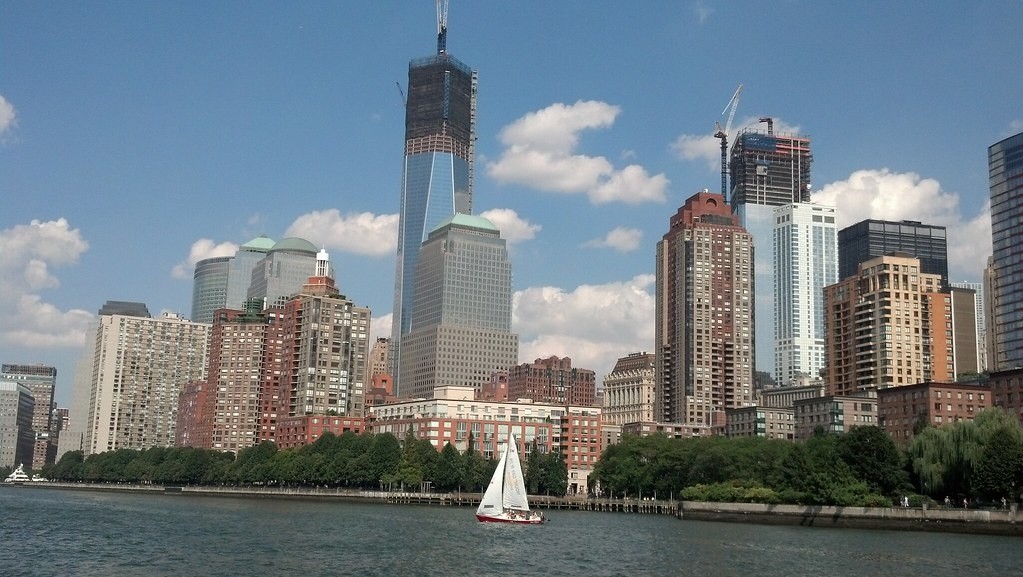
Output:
[945,495,1007,510]
[900,494,909,507]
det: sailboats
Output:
[475,433,549,525]
